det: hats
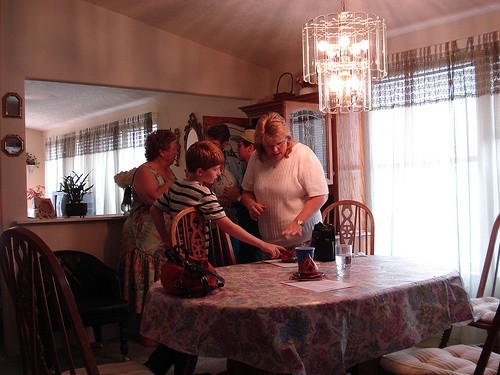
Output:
[231,129,257,144]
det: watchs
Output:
[293,219,304,227]
[237,195,242,204]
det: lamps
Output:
[301,0,391,113]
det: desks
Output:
[139,255,469,375]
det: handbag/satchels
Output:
[161,243,226,295]
[310,222,335,262]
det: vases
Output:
[121,190,134,214]
[28,165,34,173]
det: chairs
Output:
[0,226,156,375]
[379,215,500,375]
[321,200,375,255]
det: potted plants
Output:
[58,170,95,218]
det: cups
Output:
[295,246,315,265]
[334,244,352,278]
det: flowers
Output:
[26,153,42,168]
[114,168,141,211]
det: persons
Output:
[121,128,181,348]
[145,139,287,375]
[198,122,264,267]
[238,111,329,263]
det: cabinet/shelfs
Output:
[202,92,365,254]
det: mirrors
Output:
[2,134,24,158]
[2,92,22,118]
[23,78,254,222]
[184,112,203,155]
